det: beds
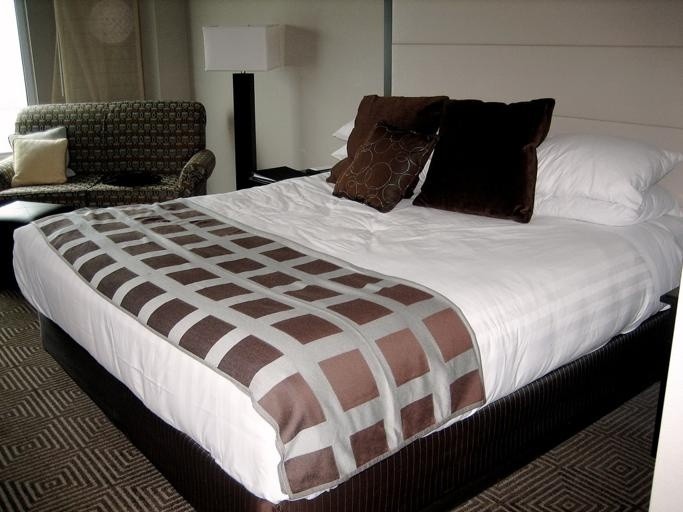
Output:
[11,146,683,512]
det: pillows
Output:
[330,123,441,213]
[8,127,76,188]
[412,96,556,225]
[326,95,450,185]
[330,145,432,194]
[535,132,681,208]
[332,118,440,160]
[531,179,681,227]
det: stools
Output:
[1,200,66,290]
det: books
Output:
[251,166,308,184]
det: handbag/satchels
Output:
[102,170,163,187]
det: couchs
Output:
[0,101,216,206]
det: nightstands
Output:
[249,169,332,187]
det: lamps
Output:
[203,24,285,191]
